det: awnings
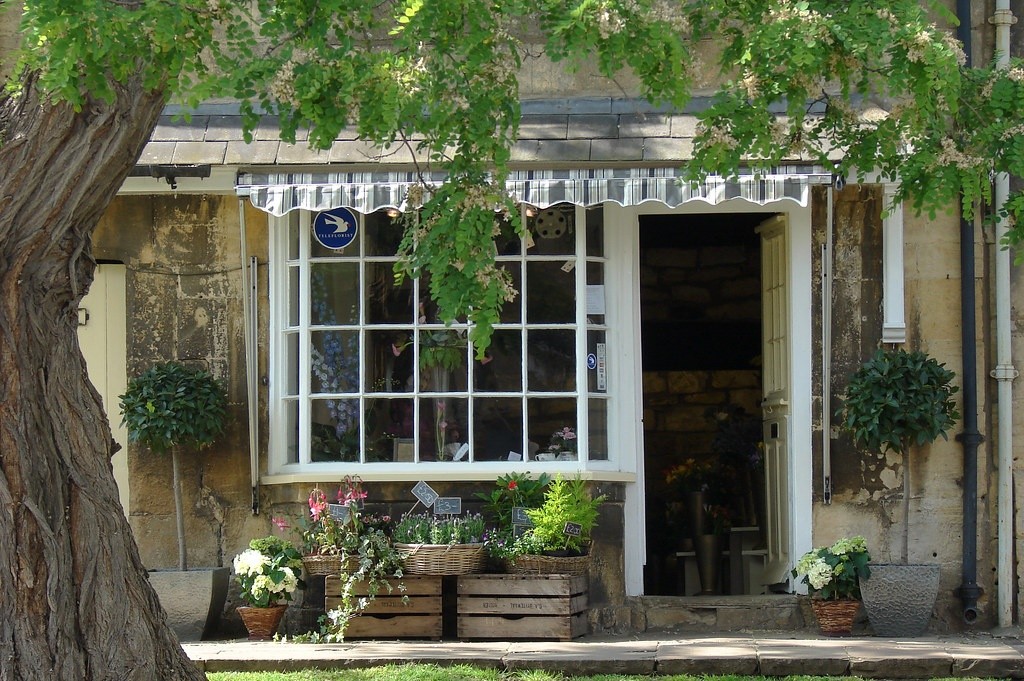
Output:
[234,164,849,217]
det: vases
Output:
[724,470,759,528]
[300,554,369,574]
[811,592,862,637]
[687,492,727,596]
[391,542,490,576]
[238,606,289,642]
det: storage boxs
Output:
[457,571,590,641]
[324,573,444,641]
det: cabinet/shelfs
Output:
[677,526,766,596]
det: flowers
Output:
[273,473,552,560]
[701,503,735,535]
[662,458,733,493]
[232,536,307,608]
[790,536,874,599]
[707,408,765,472]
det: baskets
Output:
[809,598,861,637]
[502,539,595,576]
[302,554,379,577]
[392,542,491,575]
[236,602,289,640]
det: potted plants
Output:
[655,504,693,552]
[835,348,960,636]
[476,473,609,573]
[118,362,231,641]
